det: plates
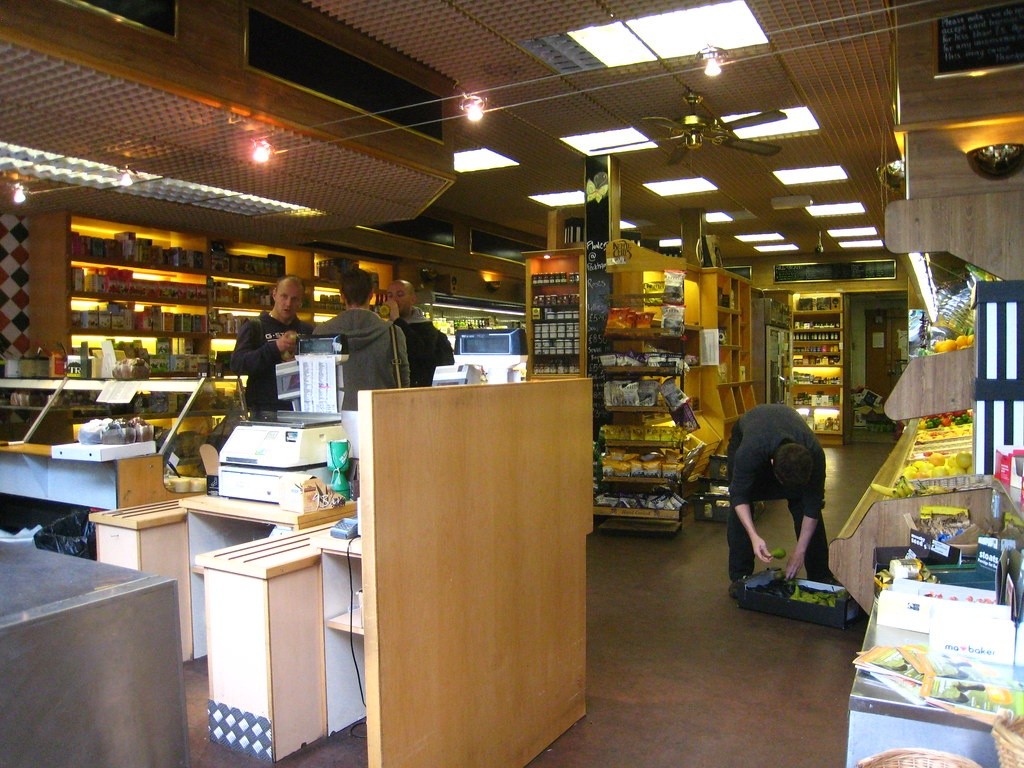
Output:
[534,359,580,374]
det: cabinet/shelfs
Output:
[177,495,365,743]
[28,211,400,478]
[522,240,795,539]
[793,292,851,445]
[830,191,1024,614]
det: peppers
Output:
[917,410,974,428]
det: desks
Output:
[846,568,1024,768]
[0,541,191,768]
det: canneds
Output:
[792,332,839,340]
[314,295,346,310]
[532,272,580,355]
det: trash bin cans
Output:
[34,510,96,561]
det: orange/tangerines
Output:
[934,334,974,354]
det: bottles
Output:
[740,361,745,381]
[817,417,840,431]
[793,333,839,352]
[432,318,447,334]
[239,287,273,308]
[447,319,488,335]
[281,330,296,362]
[422,312,430,319]
[379,304,390,317]
[793,391,812,405]
[816,392,839,405]
[374,292,387,313]
[793,371,841,385]
[793,355,839,365]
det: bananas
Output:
[869,476,945,497]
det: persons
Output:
[230,274,315,416]
[384,278,455,388]
[311,268,411,414]
[726,404,844,600]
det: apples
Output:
[901,453,974,480]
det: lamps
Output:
[876,160,906,195]
[967,143,1024,180]
[252,139,276,163]
[459,91,488,122]
[486,281,502,291]
[694,44,728,76]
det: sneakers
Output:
[819,577,845,588]
[728,576,745,596]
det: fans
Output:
[643,94,787,167]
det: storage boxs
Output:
[995,445,1024,507]
[66,390,193,414]
[211,252,286,276]
[71,301,209,333]
[214,281,274,307]
[698,476,731,493]
[72,266,207,301]
[73,337,208,373]
[217,351,233,371]
[51,441,156,462]
[854,388,894,433]
[873,513,1015,665]
[318,258,379,288]
[691,494,731,521]
[72,231,203,268]
[219,314,260,334]
[737,567,861,628]
[710,454,731,482]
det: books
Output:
[852,613,1016,726]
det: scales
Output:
[217,411,354,472]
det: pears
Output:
[789,583,844,607]
